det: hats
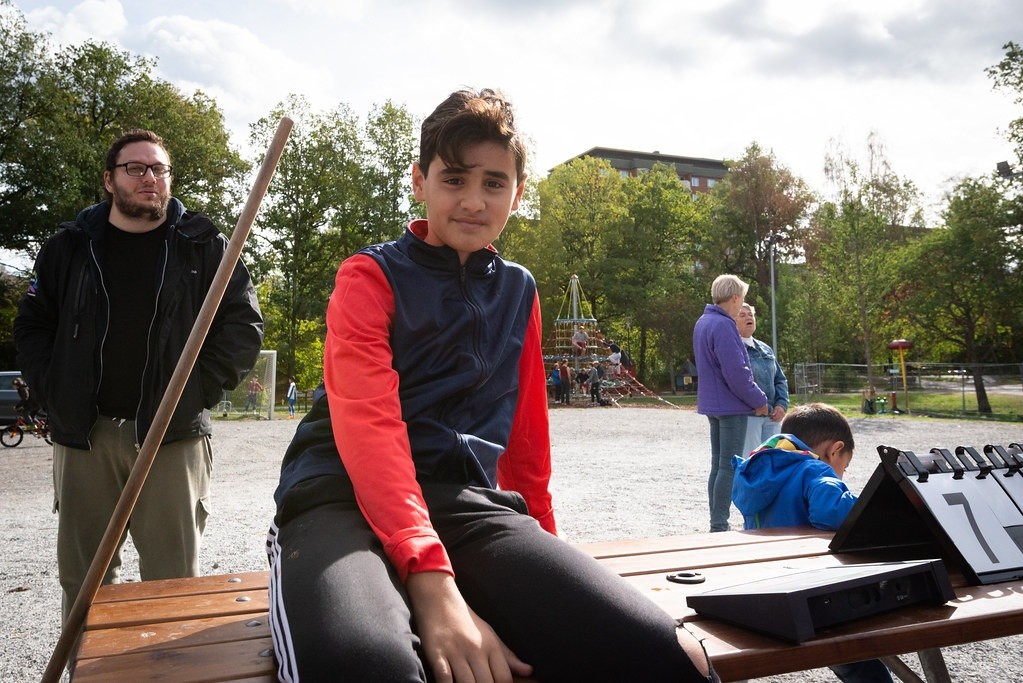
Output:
[593,361,600,364]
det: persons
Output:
[286,376,297,419]
[14,129,265,683]
[266,88,727,683]
[11,376,45,431]
[730,402,893,683]
[692,273,769,533]
[551,325,622,407]
[735,301,790,460]
[243,374,264,415]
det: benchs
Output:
[74,528,1023,683]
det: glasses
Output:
[114,162,173,177]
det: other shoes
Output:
[253,410,257,414]
[566,404,572,406]
[287,415,294,419]
[26,425,38,430]
[588,402,600,406]
[243,411,248,415]
[562,403,567,405]
[555,401,560,403]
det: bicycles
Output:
[0,407,53,446]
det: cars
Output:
[0,371,49,426]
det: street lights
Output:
[764,233,783,358]
